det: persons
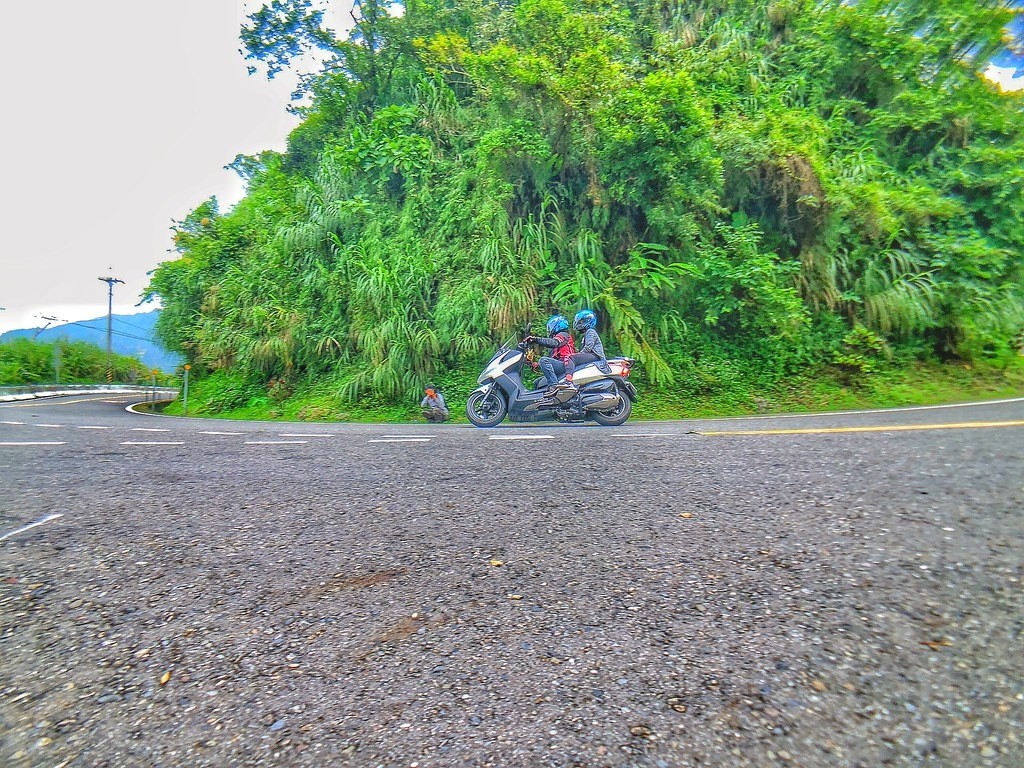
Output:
[522,314,574,398]
[420,385,450,425]
[556,310,612,389]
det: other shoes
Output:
[543,385,559,398]
[429,419,442,423]
[556,381,573,389]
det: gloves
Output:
[523,336,536,346]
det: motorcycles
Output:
[466,322,637,427]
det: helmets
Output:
[573,311,597,333]
[546,314,569,336]
